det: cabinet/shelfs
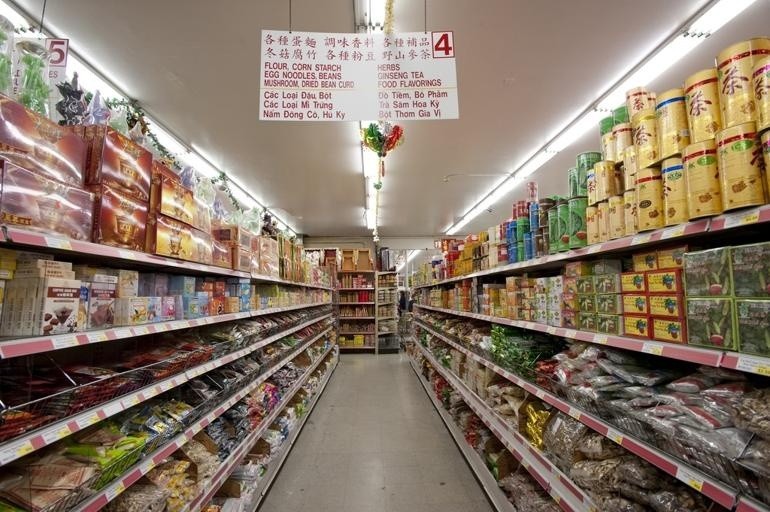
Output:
[338,270,401,355]
